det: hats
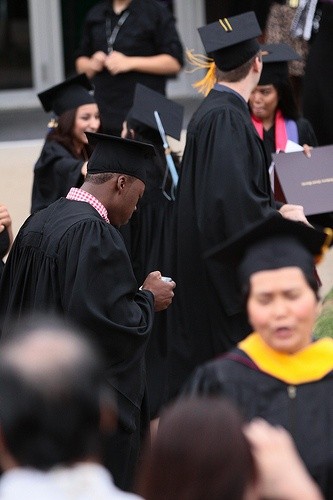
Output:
[257,41,303,80]
[38,72,99,117]
[130,83,186,188]
[203,206,333,315]
[184,11,262,97]
[83,130,159,185]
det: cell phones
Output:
[139,277,172,290]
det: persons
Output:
[177,13,314,395]
[261,0,333,146]
[0,312,151,500]
[134,397,325,500]
[111,83,186,435]
[246,42,333,265]
[0,131,177,494]
[74,0,184,138]
[29,73,101,214]
[175,215,333,499]
[0,204,13,321]
